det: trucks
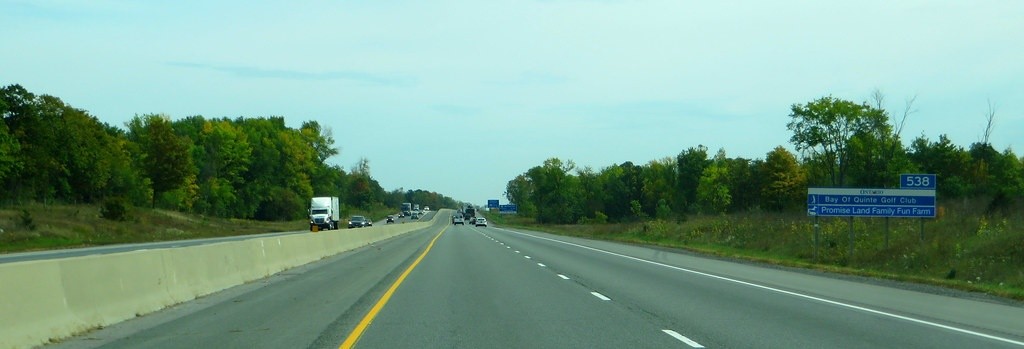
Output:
[413,203,419,211]
[401,202,411,216]
[462,206,475,220]
[309,197,340,231]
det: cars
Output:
[424,205,430,211]
[452,212,488,227]
[348,215,372,229]
[398,212,405,218]
[386,215,395,223]
[411,211,419,219]
